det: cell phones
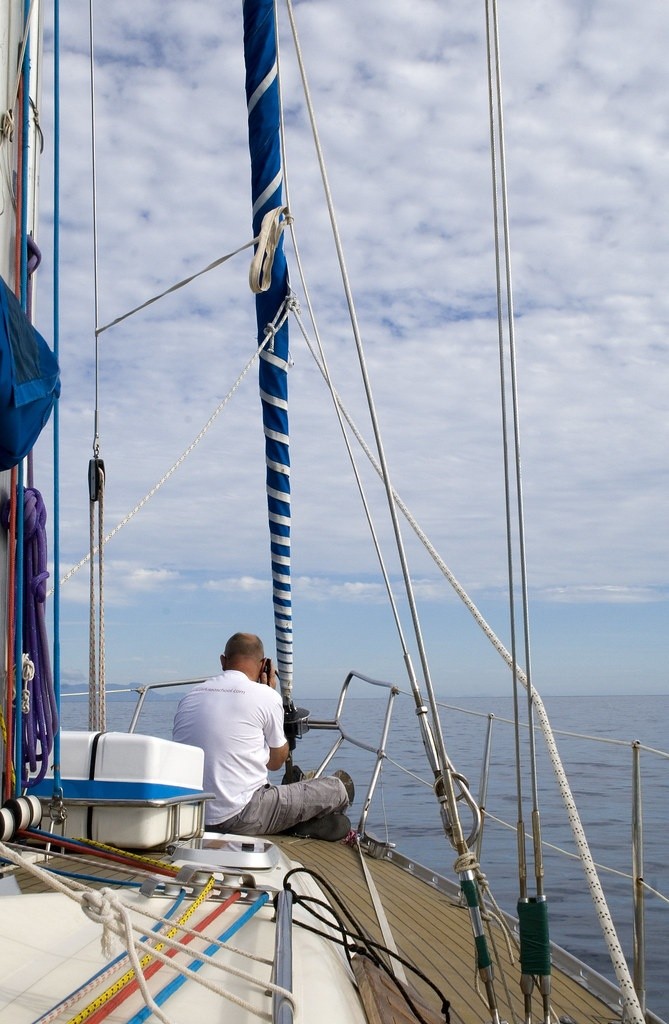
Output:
[262,659,270,685]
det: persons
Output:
[169,630,351,842]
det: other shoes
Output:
[289,814,352,842]
